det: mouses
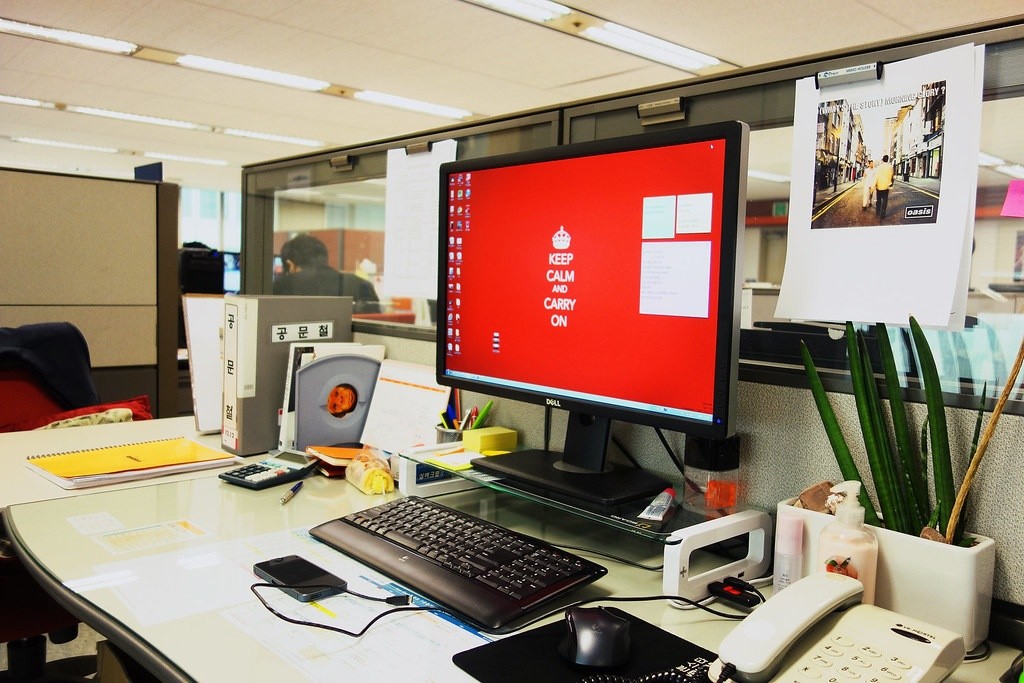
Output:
[555,606,633,666]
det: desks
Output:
[0,415,1024,683]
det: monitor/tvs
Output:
[433,118,750,510]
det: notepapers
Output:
[462,425,517,455]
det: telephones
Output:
[705,574,967,683]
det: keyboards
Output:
[308,494,609,629]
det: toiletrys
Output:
[814,480,878,605]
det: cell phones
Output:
[253,555,347,601]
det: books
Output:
[24,437,235,490]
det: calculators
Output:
[218,450,318,490]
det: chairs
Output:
[0,322,107,683]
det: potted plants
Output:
[776,313,997,653]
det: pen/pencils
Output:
[280,481,303,503]
[447,389,494,430]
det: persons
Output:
[354,249,377,283]
[863,156,894,219]
[273,235,381,314]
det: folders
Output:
[222,293,353,455]
